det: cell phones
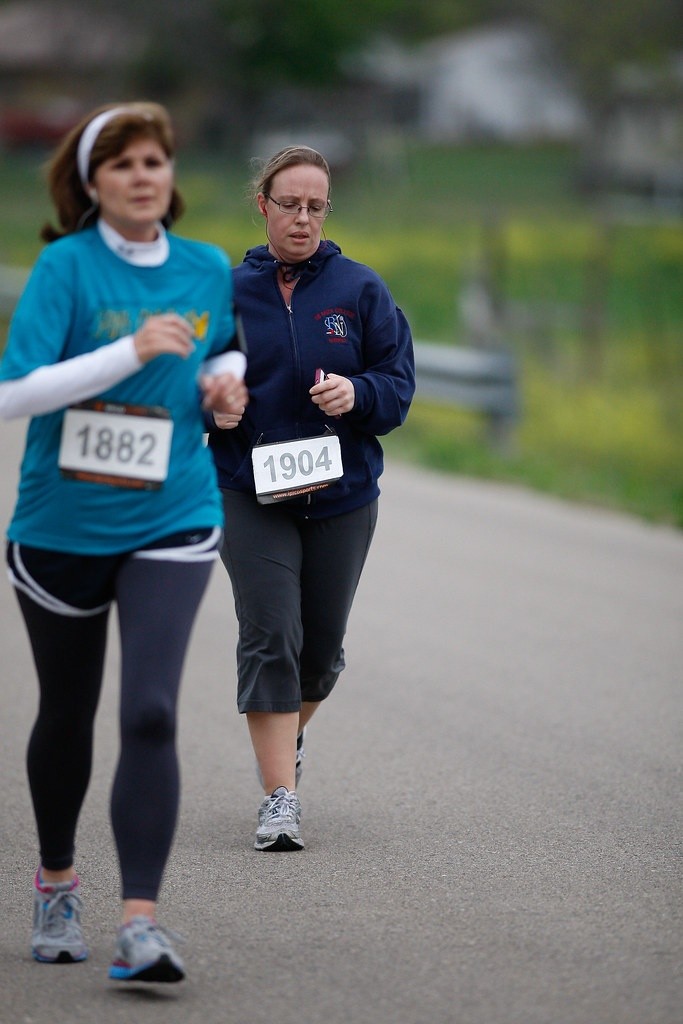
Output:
[314,369,342,420]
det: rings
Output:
[225,394,236,405]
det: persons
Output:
[197,146,417,855]
[0,103,233,985]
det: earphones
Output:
[89,188,99,202]
[261,208,268,216]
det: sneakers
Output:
[253,785,305,851]
[30,864,89,962]
[256,726,305,792]
[108,915,186,982]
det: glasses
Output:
[265,192,334,218]
